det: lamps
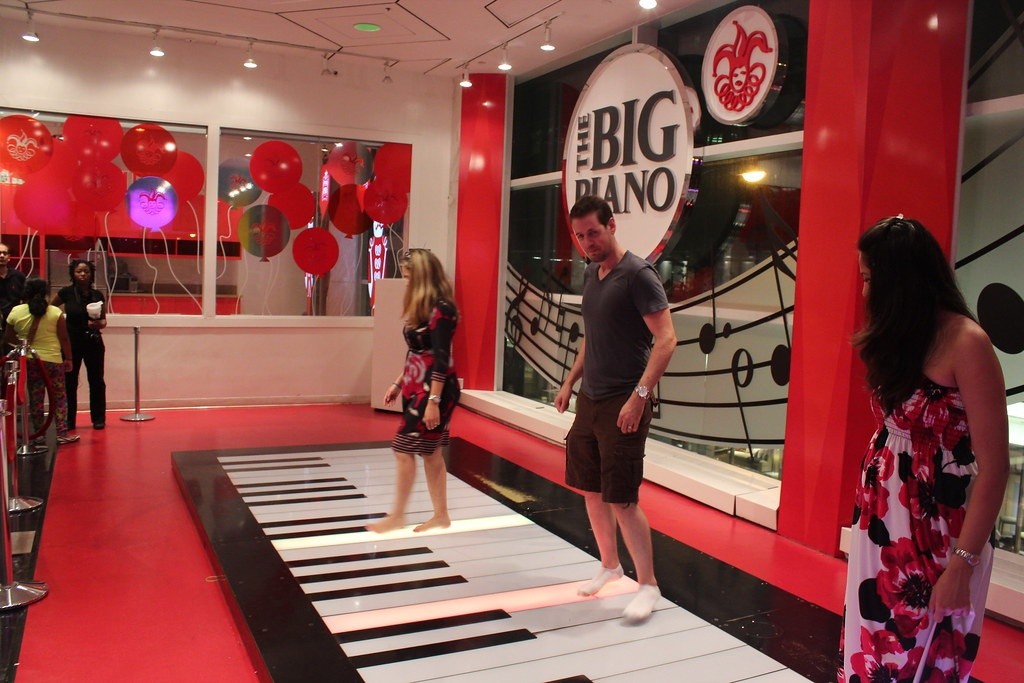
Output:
[381,60,393,84]
[540,20,555,51]
[319,50,338,78]
[22,10,40,43]
[244,41,258,69]
[149,26,165,57]
[498,46,512,71]
[459,67,472,87]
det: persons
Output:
[0,244,107,448]
[554,196,678,621]
[364,248,460,535]
[835,215,1010,683]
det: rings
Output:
[628,425,633,429]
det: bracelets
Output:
[393,383,402,390]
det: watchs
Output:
[429,395,442,404]
[634,385,651,401]
[952,547,981,567]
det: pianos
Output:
[171,436,841,683]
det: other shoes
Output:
[56,433,81,444]
[31,432,48,444]
[94,423,105,430]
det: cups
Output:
[86,303,102,318]
